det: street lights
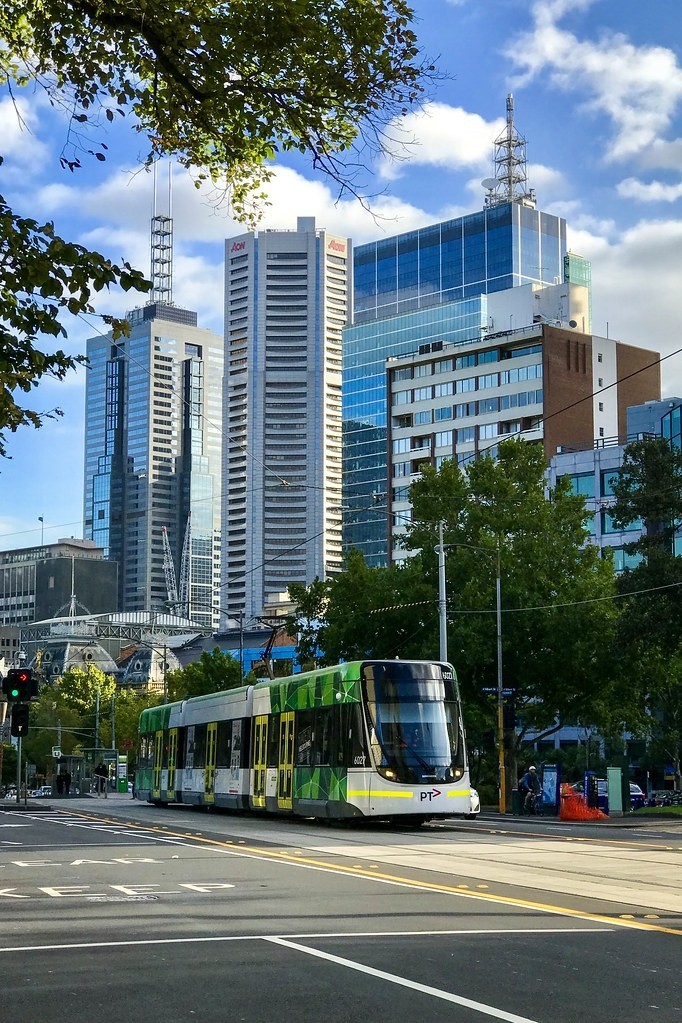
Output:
[432,544,505,812]
[164,601,243,685]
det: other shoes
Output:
[522,804,529,810]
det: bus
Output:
[134,658,471,824]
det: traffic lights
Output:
[7,675,21,700]
[12,704,28,738]
[20,669,30,701]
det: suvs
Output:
[571,778,646,816]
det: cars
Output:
[650,789,682,808]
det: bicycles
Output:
[521,788,545,818]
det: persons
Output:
[62,769,71,795]
[95,762,108,793]
[522,765,543,811]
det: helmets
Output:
[529,766,536,771]
